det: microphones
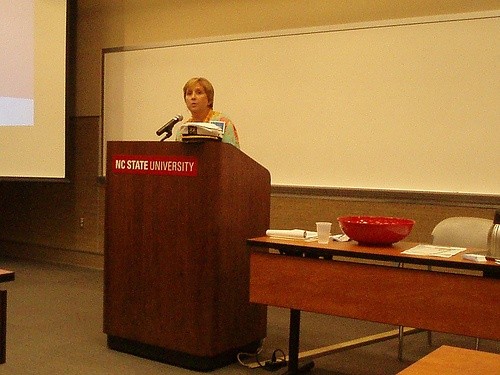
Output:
[156,114,184,136]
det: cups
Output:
[316,222,333,244]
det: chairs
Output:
[391,215,500,361]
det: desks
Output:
[244,229,500,375]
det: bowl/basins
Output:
[336,215,416,247]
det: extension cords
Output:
[265,358,287,371]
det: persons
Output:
[174,77,239,149]
